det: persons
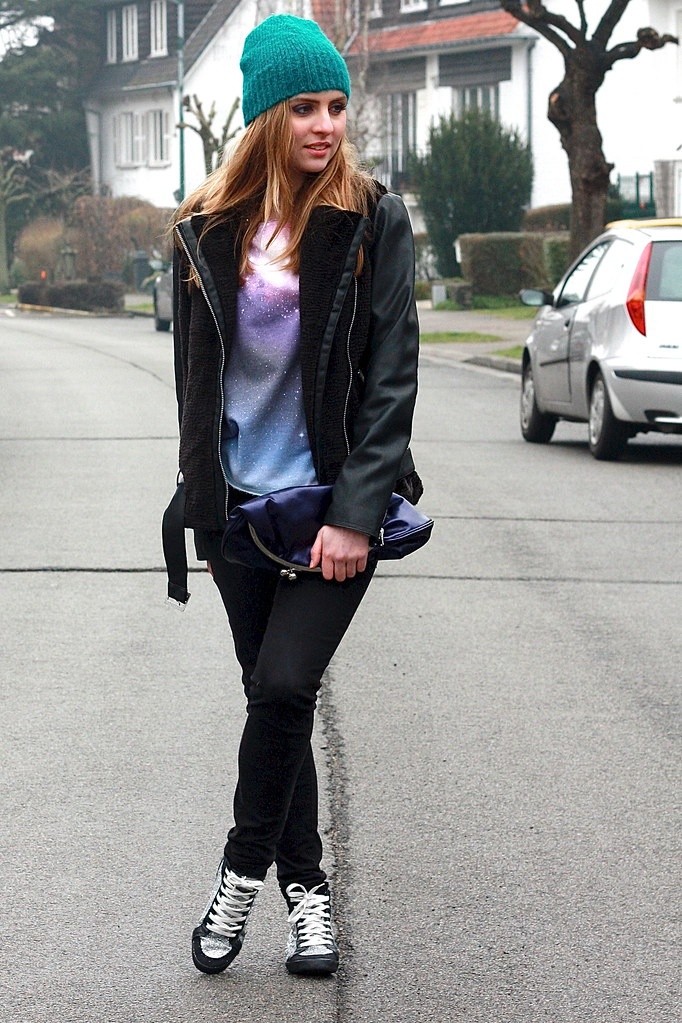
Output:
[163,13,421,978]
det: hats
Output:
[240,12,352,129]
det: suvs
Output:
[518,215,682,461]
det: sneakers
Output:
[280,881,341,975]
[192,854,266,973]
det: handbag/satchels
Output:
[221,483,434,581]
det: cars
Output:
[153,260,174,333]
